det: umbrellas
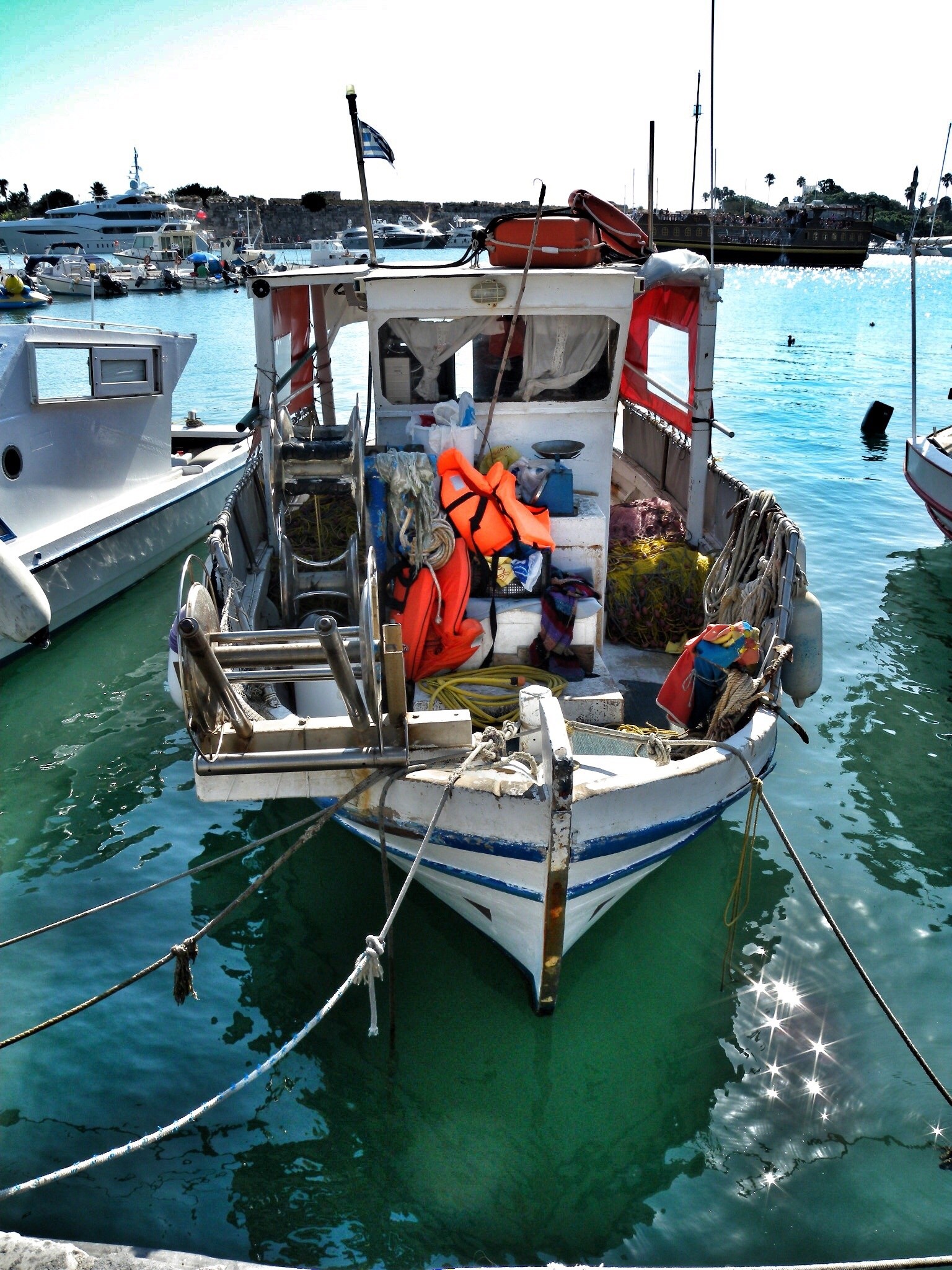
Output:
[187,251,219,263]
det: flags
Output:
[196,210,207,219]
[360,121,395,164]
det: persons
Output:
[631,207,856,245]
[193,261,223,277]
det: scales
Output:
[529,440,585,516]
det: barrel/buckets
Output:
[294,663,368,720]
[412,423,478,468]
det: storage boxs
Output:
[468,594,597,654]
[470,546,551,598]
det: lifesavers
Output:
[567,188,659,257]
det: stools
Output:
[186,444,238,468]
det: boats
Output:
[904,241,952,541]
[866,121,952,258]
[0,314,262,669]
[0,146,221,269]
[163,0,831,1019]
[0,193,490,312]
